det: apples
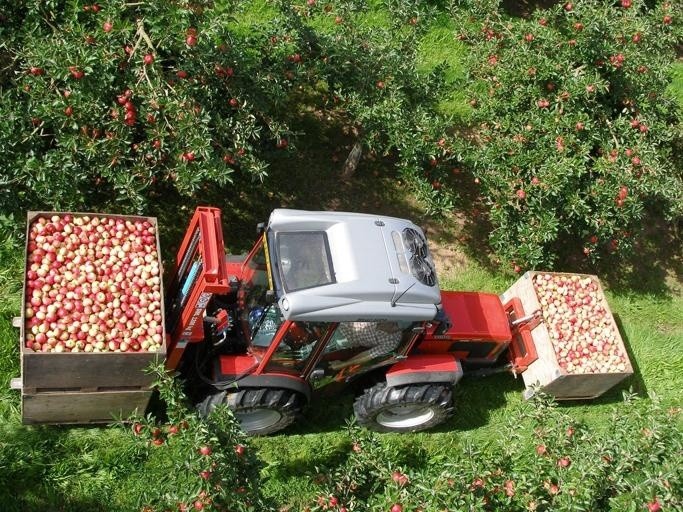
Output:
[135,424,671,512]
[410,0,673,255]
[511,262,626,374]
[25,0,384,185]
[25,214,163,353]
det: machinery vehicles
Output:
[165,201,515,440]
